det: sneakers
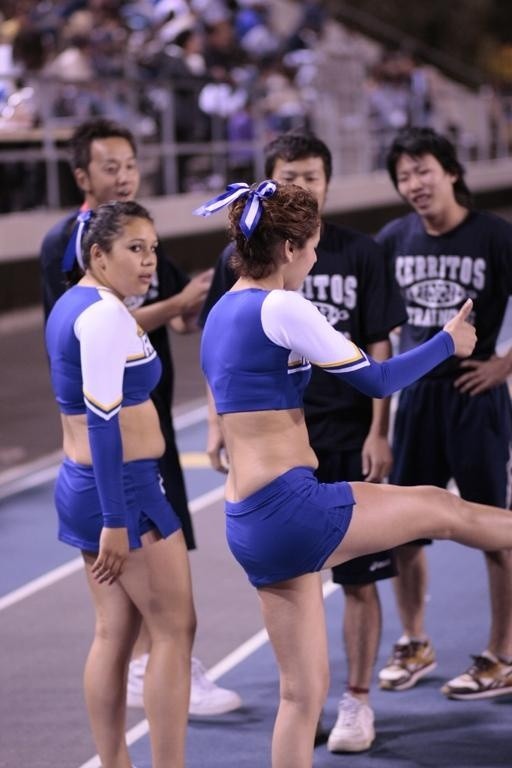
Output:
[328,690,376,754]
[379,636,437,690]
[440,650,512,701]
[122,652,241,716]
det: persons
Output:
[46,201,197,767]
[198,179,511,768]
[37,117,242,718]
[0,0,511,217]
[370,127,512,700]
[199,131,400,753]
